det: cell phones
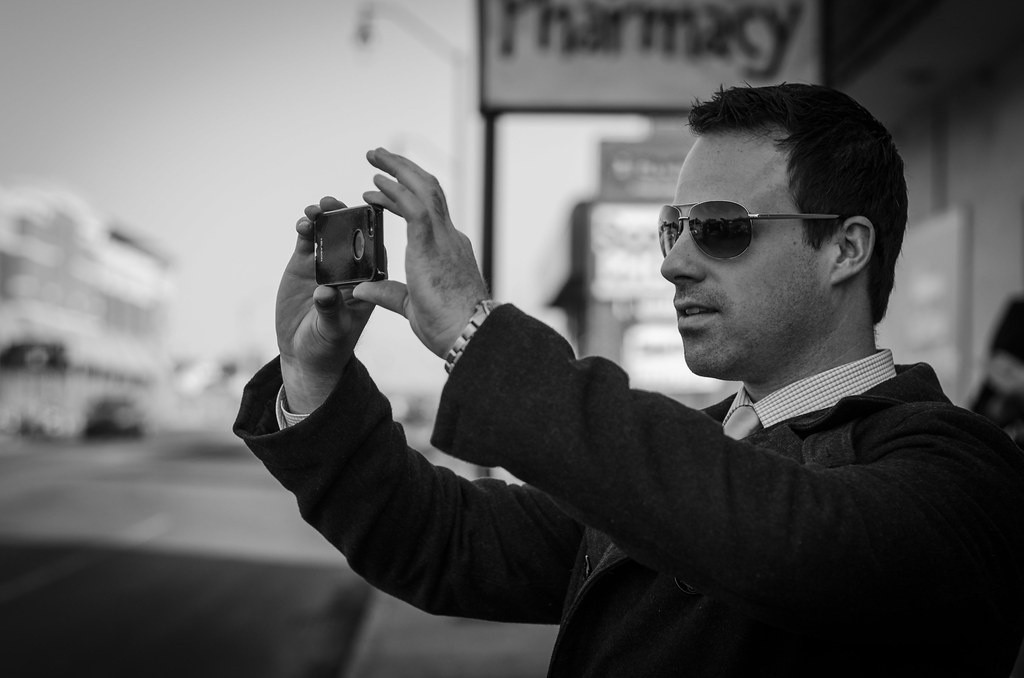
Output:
[312,203,385,286]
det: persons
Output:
[232,83,1024,678]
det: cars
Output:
[386,384,442,458]
[136,351,271,461]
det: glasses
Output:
[658,201,843,262]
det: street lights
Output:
[354,0,468,248]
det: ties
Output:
[723,406,758,442]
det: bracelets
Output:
[444,299,502,375]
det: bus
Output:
[0,340,154,439]
